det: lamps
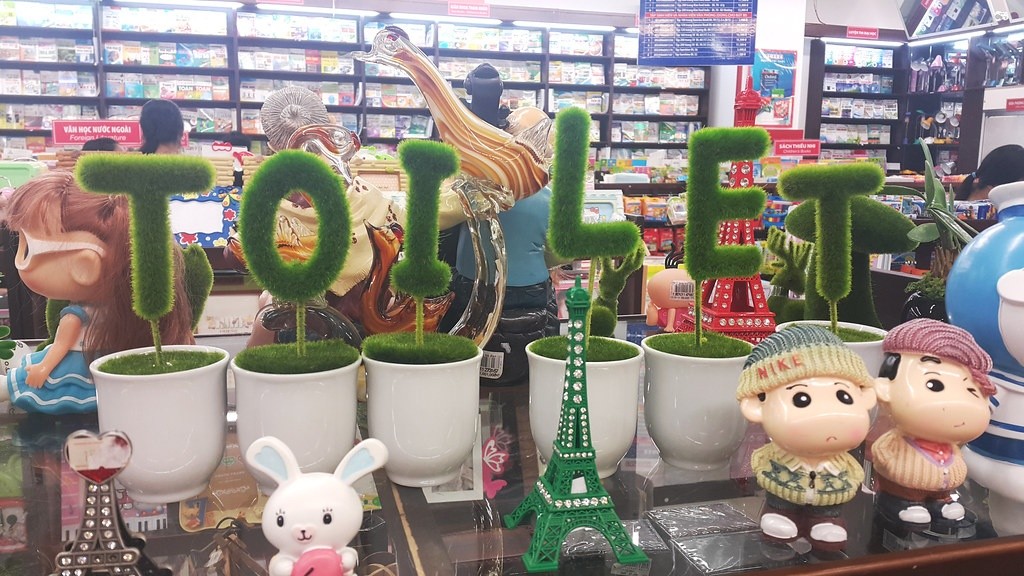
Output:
[977,37,1024,88]
[905,51,967,176]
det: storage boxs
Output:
[624,196,685,255]
[758,194,792,227]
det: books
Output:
[0,1,704,255]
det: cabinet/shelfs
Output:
[596,181,997,314]
[804,37,907,172]
[903,39,987,176]
[0,0,710,168]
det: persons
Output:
[956,144,1024,201]
[870,320,998,500]
[6,515,25,543]
[737,324,878,509]
[0,170,197,414]
[82,137,125,153]
[138,97,183,154]
[436,186,556,340]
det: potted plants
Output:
[774,160,889,379]
[638,127,768,471]
[359,138,483,489]
[73,150,230,504]
[229,147,361,497]
[525,106,647,479]
[882,138,981,324]
